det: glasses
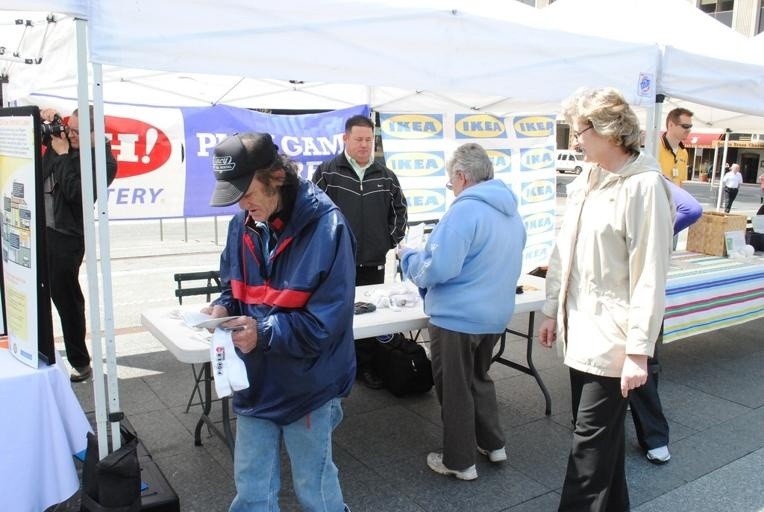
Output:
[446,179,453,190]
[62,121,79,134]
[575,125,595,139]
[679,120,693,130]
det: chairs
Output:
[405,218,438,242]
[174,271,236,438]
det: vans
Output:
[555,152,585,175]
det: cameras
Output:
[42,113,68,147]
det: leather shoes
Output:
[358,372,386,389]
[67,362,92,383]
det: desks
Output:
[662,251,764,344]
[0,347,93,512]
[141,272,552,463]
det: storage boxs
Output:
[686,211,747,257]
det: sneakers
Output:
[472,436,508,463]
[647,443,668,464]
[425,451,478,482]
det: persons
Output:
[538,86,672,512]
[397,144,527,479]
[41,105,118,382]
[312,115,407,286]
[197,132,357,512]
[721,163,744,213]
[660,109,693,190]
[628,172,702,466]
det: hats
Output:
[209,131,280,206]
